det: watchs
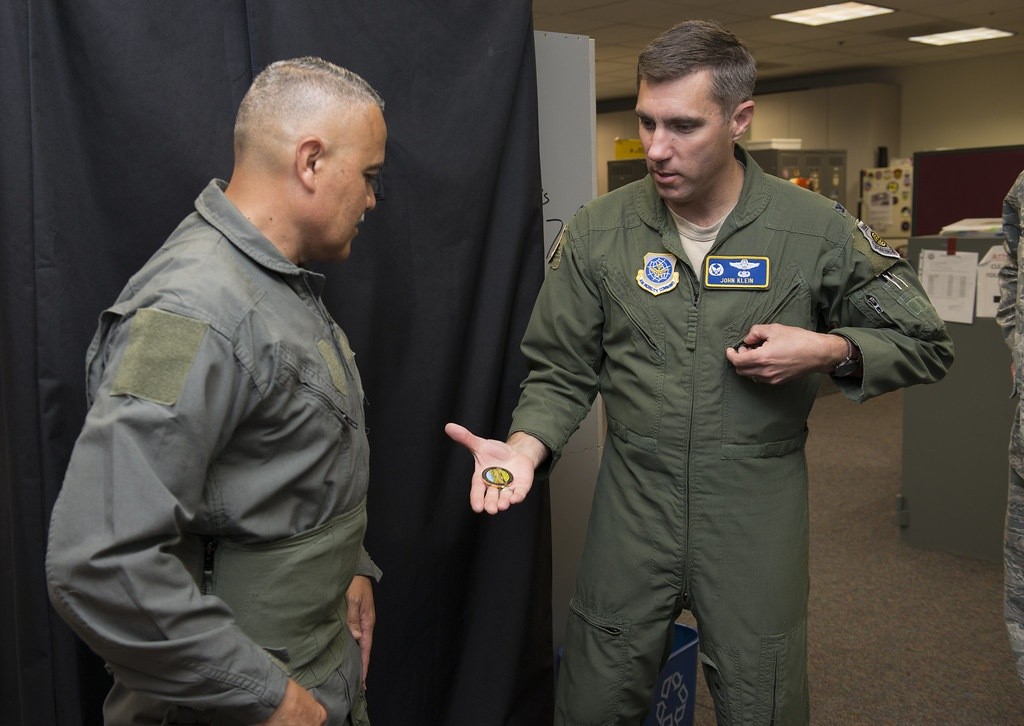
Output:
[831,335,861,379]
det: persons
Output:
[442,19,957,726]
[45,55,388,726]
[994,168,1024,685]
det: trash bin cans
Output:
[652,622,700,726]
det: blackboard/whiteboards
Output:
[858,163,913,261]
